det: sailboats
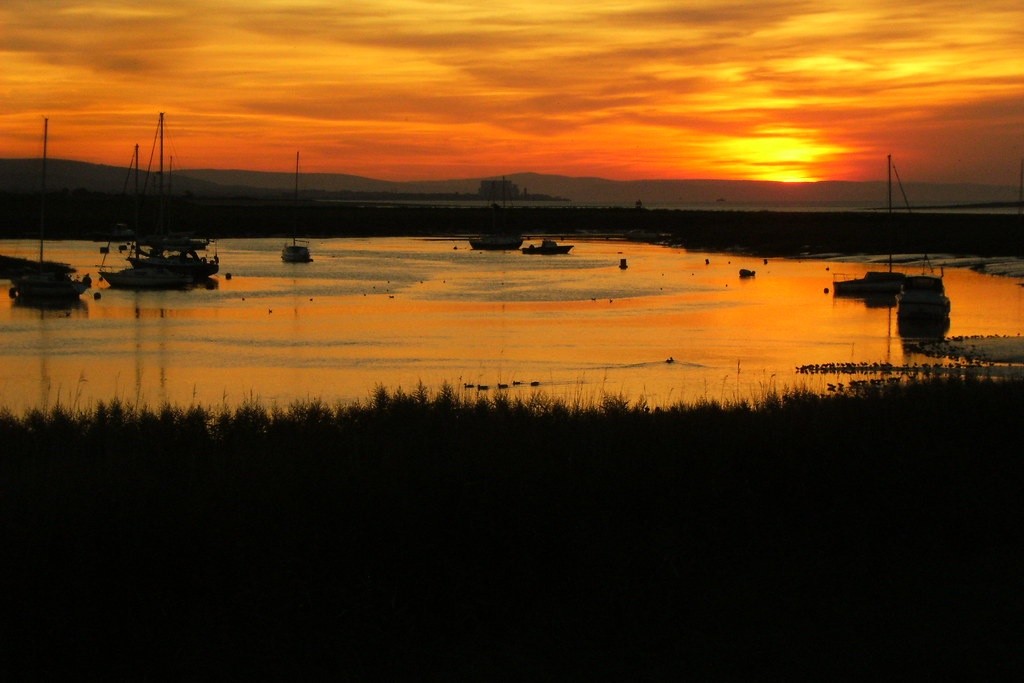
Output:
[146,155,215,252]
[126,112,219,280]
[832,154,946,296]
[468,175,524,250]
[98,144,194,289]
[8,118,94,308]
[281,152,314,262]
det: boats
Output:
[522,240,575,255]
[896,272,951,323]
